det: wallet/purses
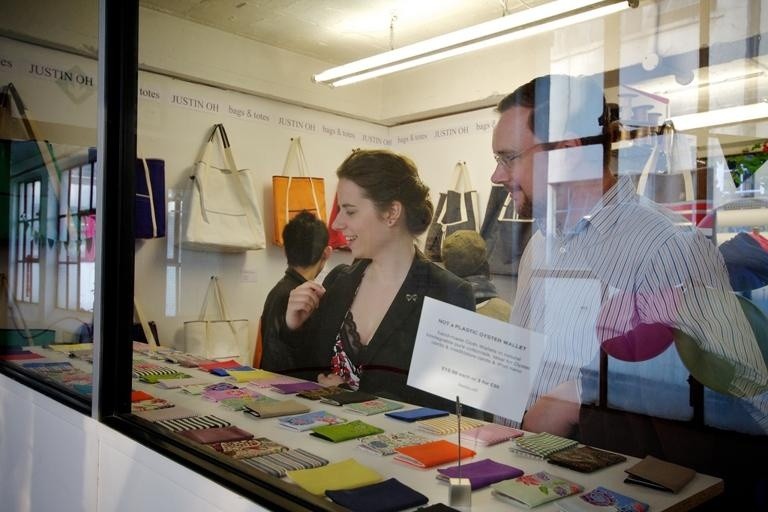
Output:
[0,340,697,511]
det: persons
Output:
[441,230,513,324]
[271,150,477,415]
[491,75,767,440]
[258,210,332,381]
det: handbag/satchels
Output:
[615,121,716,242]
[272,175,328,248]
[183,319,251,360]
[133,317,161,345]
[1,328,55,345]
[425,190,480,263]
[0,135,82,244]
[176,159,268,253]
[134,158,166,239]
[329,189,348,250]
[479,186,532,277]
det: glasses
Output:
[492,140,559,170]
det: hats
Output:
[441,230,487,275]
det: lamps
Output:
[311,1,640,90]
